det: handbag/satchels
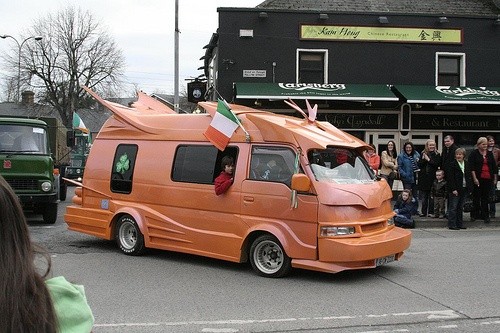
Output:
[394,215,415,228]
[488,183,500,203]
[392,168,404,191]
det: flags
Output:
[204,99,241,153]
[72,111,90,137]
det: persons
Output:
[312,144,371,184]
[214,155,234,196]
[247,158,291,182]
[0,173,96,333]
[364,135,500,229]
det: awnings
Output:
[393,84,500,105]
[234,78,400,102]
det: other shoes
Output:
[449,225,459,230]
[434,213,439,217]
[457,224,467,229]
[419,213,427,217]
[428,214,434,217]
[439,214,445,218]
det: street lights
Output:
[0,34,43,103]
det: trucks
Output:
[0,114,72,224]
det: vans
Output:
[61,84,413,277]
